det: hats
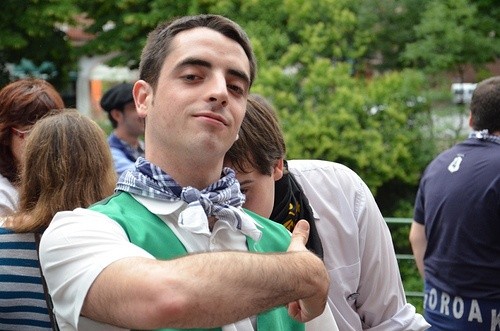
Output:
[100,82,135,110]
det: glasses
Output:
[9,126,33,141]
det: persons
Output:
[223,94,432,331]
[0,78,64,218]
[0,109,118,331]
[39,14,339,331]
[409,76,500,331]
[100,82,145,175]
